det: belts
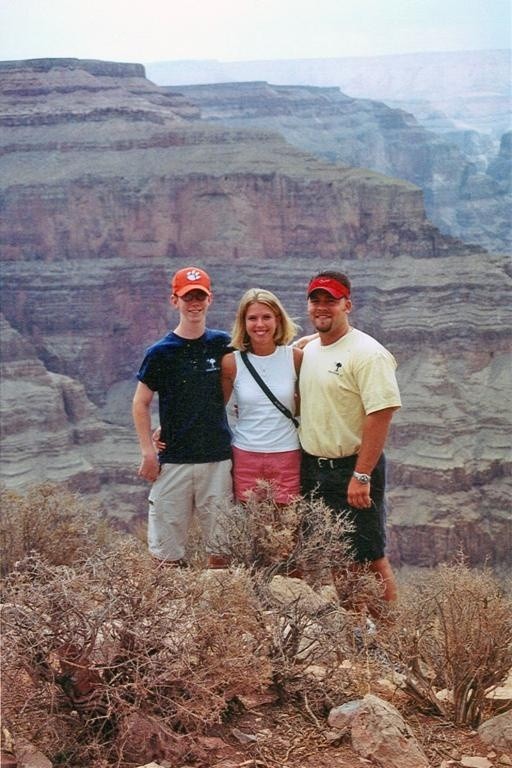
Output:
[302,450,357,469]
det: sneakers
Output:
[353,618,379,649]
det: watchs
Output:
[352,471,371,484]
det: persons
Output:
[153,288,304,579]
[133,267,239,569]
[234,271,401,635]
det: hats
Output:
[306,275,351,301]
[172,266,211,297]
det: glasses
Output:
[182,292,207,302]
